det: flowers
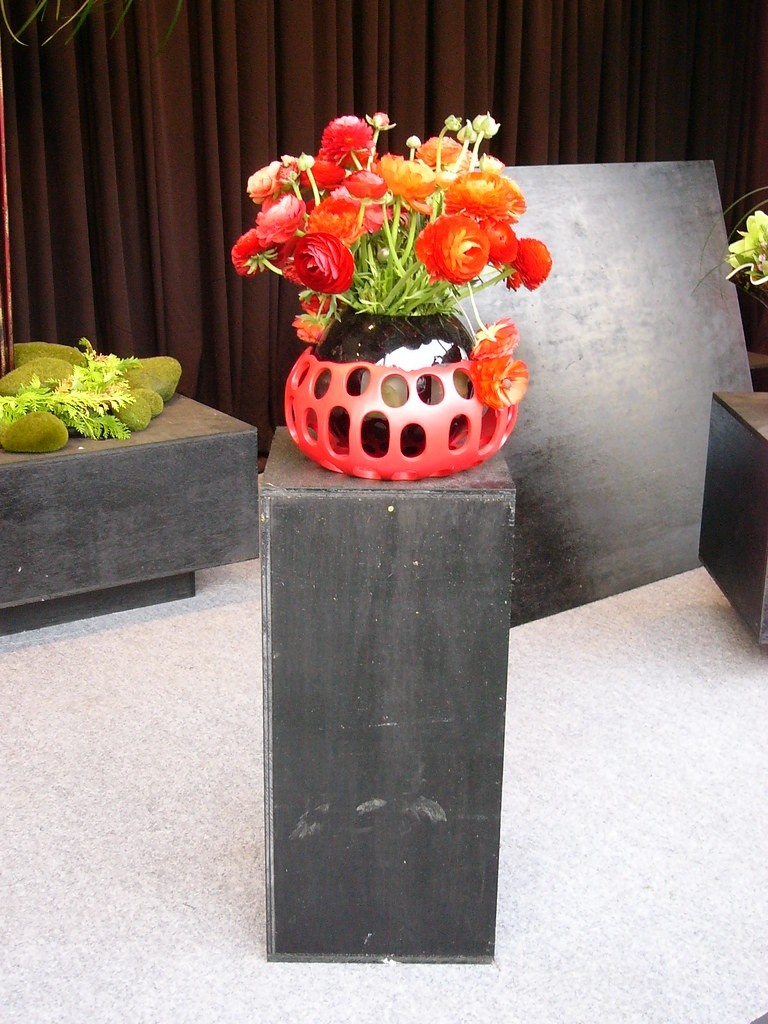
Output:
[690,185,768,304]
[228,109,553,410]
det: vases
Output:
[283,304,517,479]
